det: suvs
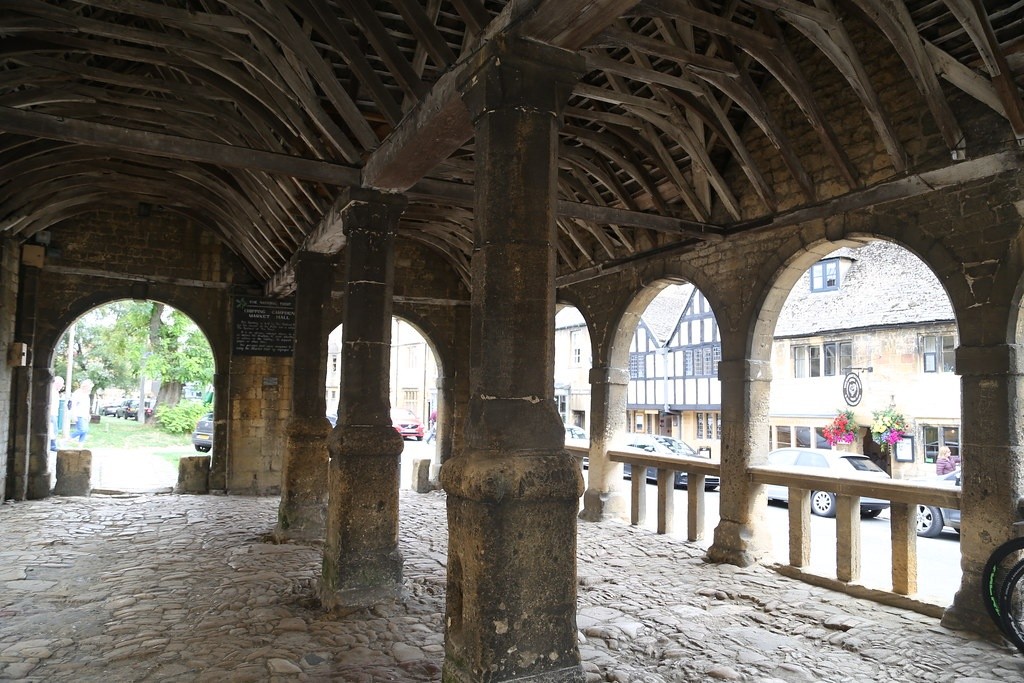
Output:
[761,447,894,520]
[114,399,150,420]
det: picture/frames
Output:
[894,435,915,463]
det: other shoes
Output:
[426,440,429,444]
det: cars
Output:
[619,433,721,491]
[564,424,592,468]
[99,400,121,417]
[908,467,962,538]
[390,405,425,442]
[192,413,215,455]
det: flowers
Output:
[871,406,909,452]
[821,410,862,446]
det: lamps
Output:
[36,230,52,245]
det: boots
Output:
[51,440,58,451]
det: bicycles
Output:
[980,536,1024,650]
[999,557,1024,652]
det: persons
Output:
[58,379,94,449]
[49,376,64,451]
[425,410,437,445]
[936,446,961,476]
